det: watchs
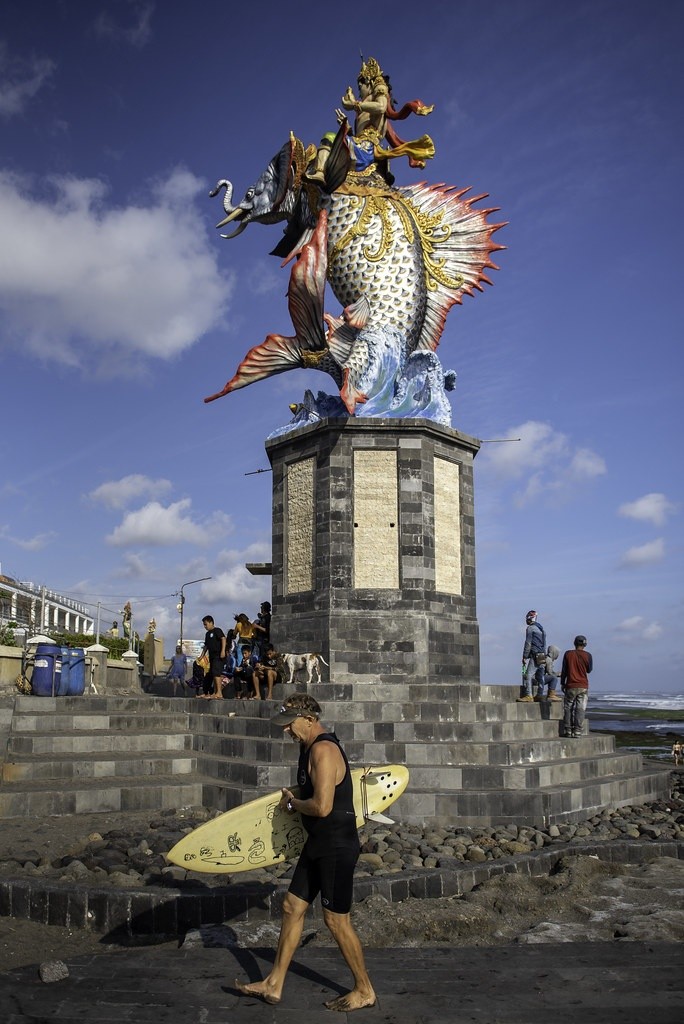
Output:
[286,797,294,812]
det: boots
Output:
[547,689,562,702]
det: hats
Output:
[260,602,272,611]
[526,610,538,621]
[268,693,321,726]
[574,635,587,647]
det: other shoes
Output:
[249,696,262,700]
[204,692,216,699]
[517,695,535,702]
[534,696,546,702]
[564,731,581,738]
[211,693,224,699]
[265,697,274,701]
[242,694,251,700]
[232,696,241,700]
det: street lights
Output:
[180,575,212,645]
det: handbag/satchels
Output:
[166,672,173,679]
[536,653,546,666]
[671,750,674,756]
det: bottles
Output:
[522,663,527,675]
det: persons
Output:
[561,634,593,737]
[516,610,562,702]
[672,740,684,767]
[193,601,284,701]
[166,644,188,696]
[234,693,377,1012]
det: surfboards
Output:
[166,764,410,875]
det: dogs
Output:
[274,652,329,685]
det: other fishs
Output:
[204,132,512,414]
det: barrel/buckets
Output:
[32,642,86,696]
